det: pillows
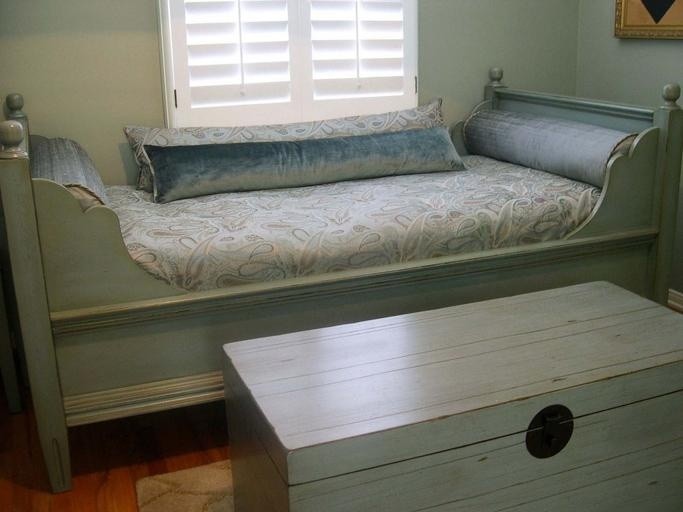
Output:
[142,126,468,203]
[28,134,107,211]
[123,99,449,191]
[466,105,637,192]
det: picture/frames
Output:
[615,0,683,41]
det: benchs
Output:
[0,66,683,494]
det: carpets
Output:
[136,459,234,512]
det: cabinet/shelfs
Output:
[221,280,683,512]
